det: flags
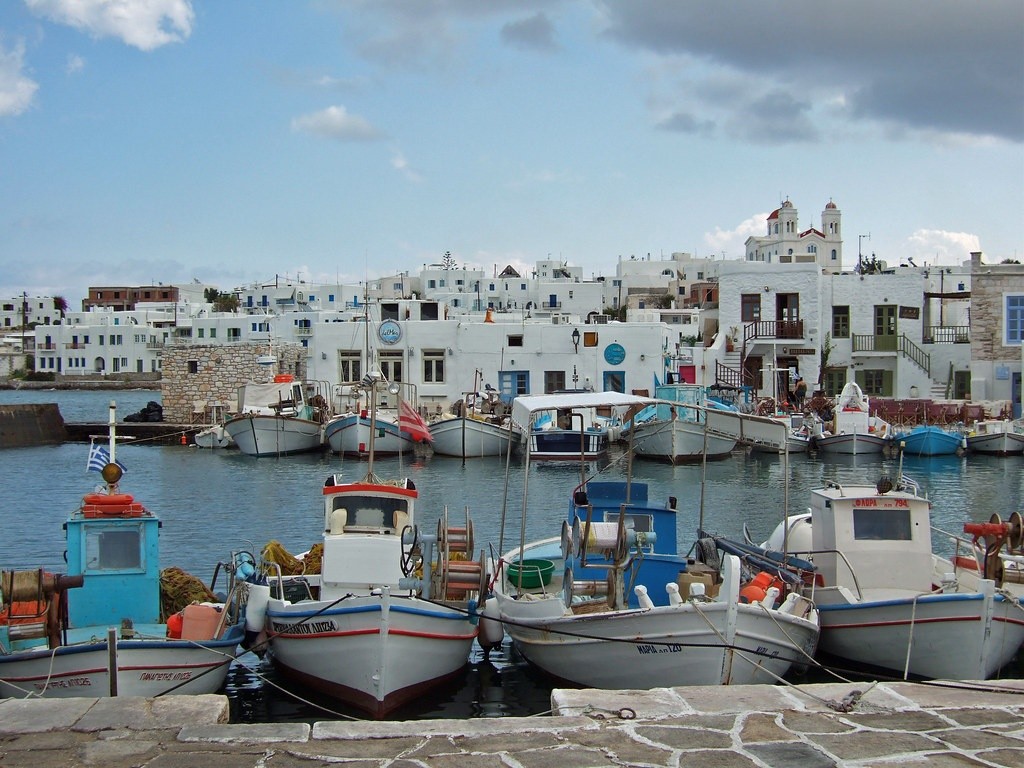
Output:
[792,367,800,380]
[398,396,435,443]
[88,442,127,479]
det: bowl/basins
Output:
[507,558,555,588]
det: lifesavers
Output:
[275,374,293,378]
[952,556,984,571]
[84,492,134,505]
[783,402,796,413]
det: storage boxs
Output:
[677,572,713,602]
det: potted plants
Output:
[965,389,971,399]
[910,386,918,398]
[725,326,737,352]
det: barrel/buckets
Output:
[166,604,225,642]
[676,570,713,605]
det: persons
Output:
[681,377,687,384]
[793,377,808,409]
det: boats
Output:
[6,274,1021,722]
